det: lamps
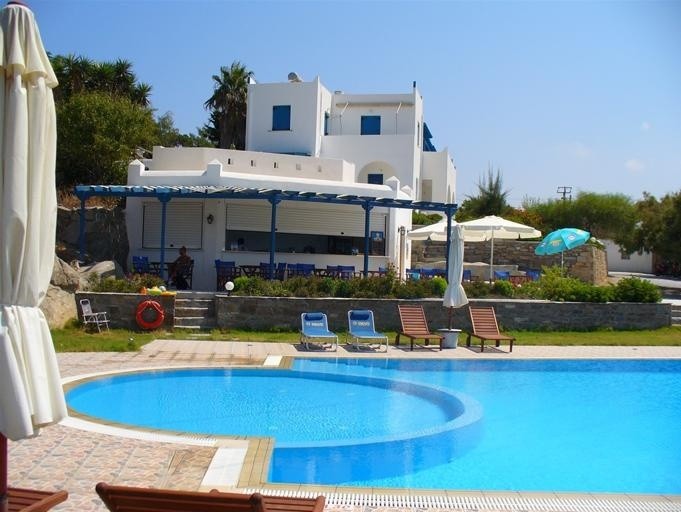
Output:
[207,214,214,224]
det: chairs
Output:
[79,298,111,335]
[7,486,68,512]
[95,482,325,512]
[299,312,338,352]
[395,304,445,352]
[467,305,516,353]
[133,256,542,292]
[346,310,389,353]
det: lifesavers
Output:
[135,301,164,330]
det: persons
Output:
[167,246,191,286]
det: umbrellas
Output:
[406,217,458,242]
[533,227,590,272]
[0,1,69,511]
[444,214,541,280]
[441,223,468,330]
